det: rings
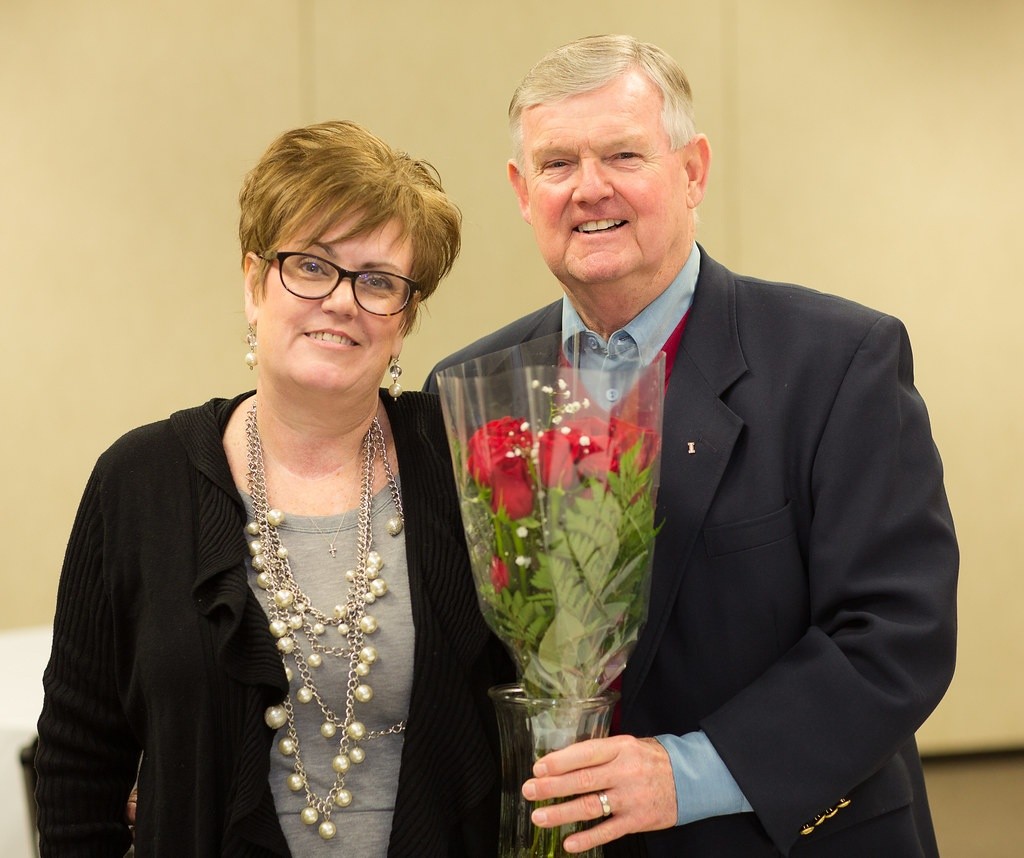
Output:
[596,791,610,816]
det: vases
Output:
[487,684,622,858]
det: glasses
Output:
[257,251,421,316]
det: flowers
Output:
[455,372,665,858]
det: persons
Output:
[420,32,957,858]
[34,119,520,858]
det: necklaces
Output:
[246,400,408,839]
